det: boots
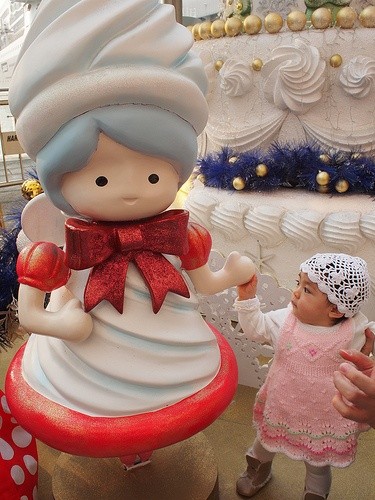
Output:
[304,486,330,500]
[236,448,276,498]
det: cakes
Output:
[173,0,375,326]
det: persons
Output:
[235,253,375,500]
[5,1,257,499]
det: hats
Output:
[300,253,370,318]
